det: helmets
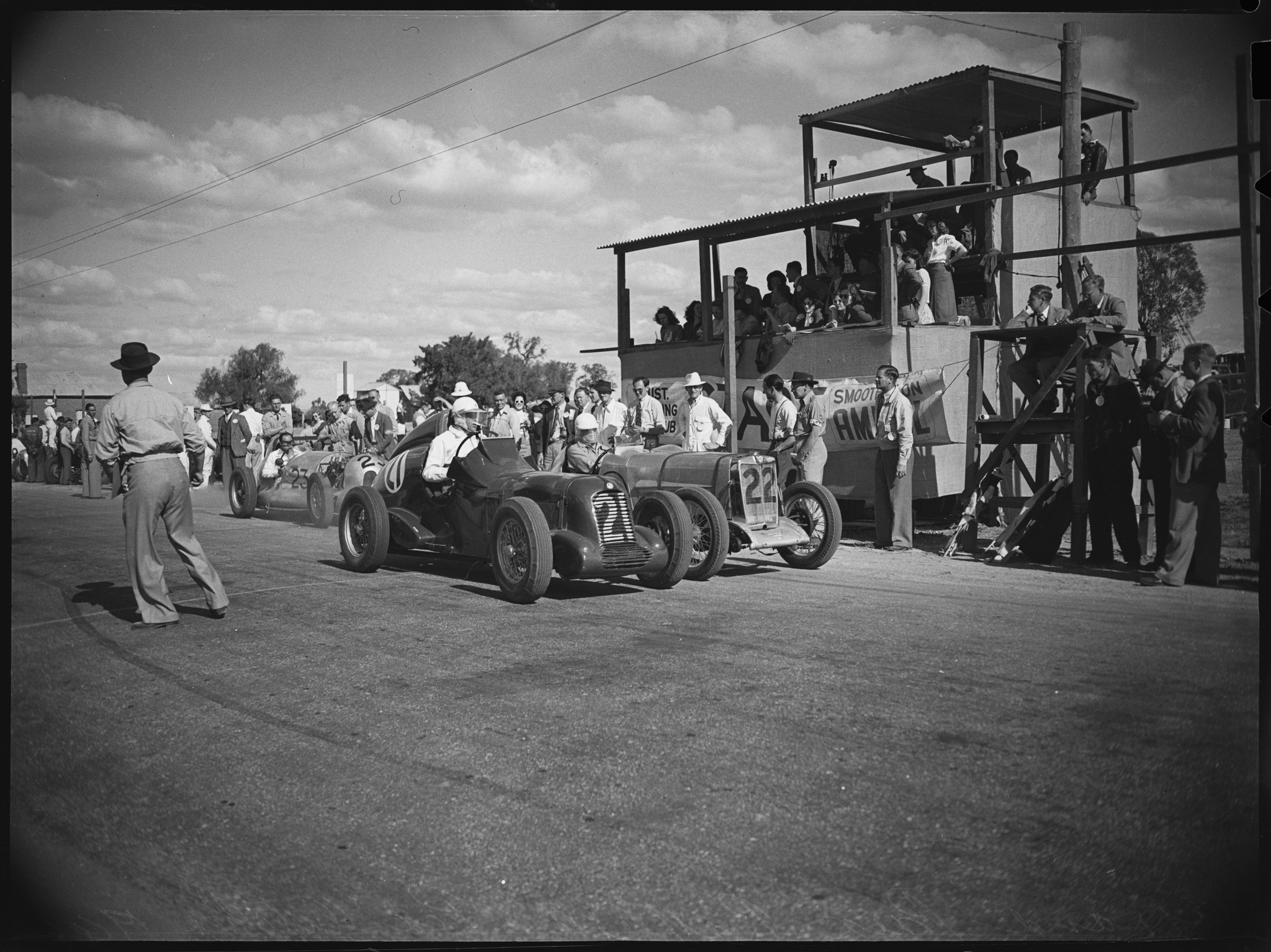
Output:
[451,397,486,416]
[574,412,599,432]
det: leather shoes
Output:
[131,602,229,629]
[1139,576,1165,585]
[867,542,912,551]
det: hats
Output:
[44,399,54,406]
[109,342,160,370]
[767,270,786,283]
[786,371,819,385]
[1137,354,1170,390]
[360,397,377,410]
[451,381,472,396]
[546,372,707,394]
[199,396,237,410]
[905,166,927,176]
[969,116,983,125]
[63,416,73,422]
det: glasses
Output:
[790,384,803,389]
[1079,289,1095,297]
[515,394,560,410]
[926,222,936,228]
[632,387,645,392]
[281,440,294,446]
[712,294,849,315]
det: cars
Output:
[227,433,384,527]
[336,417,669,599]
[12,434,109,487]
[517,444,844,582]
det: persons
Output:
[94,342,229,627]
[12,114,1254,590]
